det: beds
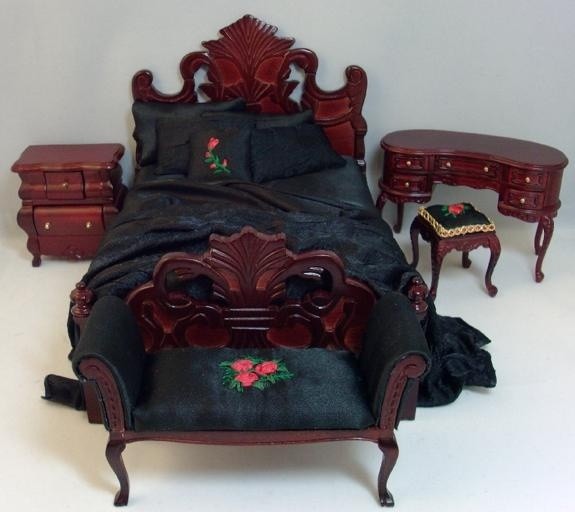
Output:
[69,16,434,421]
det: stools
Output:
[408,202,497,309]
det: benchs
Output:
[73,293,433,506]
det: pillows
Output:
[131,97,347,184]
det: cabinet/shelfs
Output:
[376,128,568,283]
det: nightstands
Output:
[11,144,125,267]
